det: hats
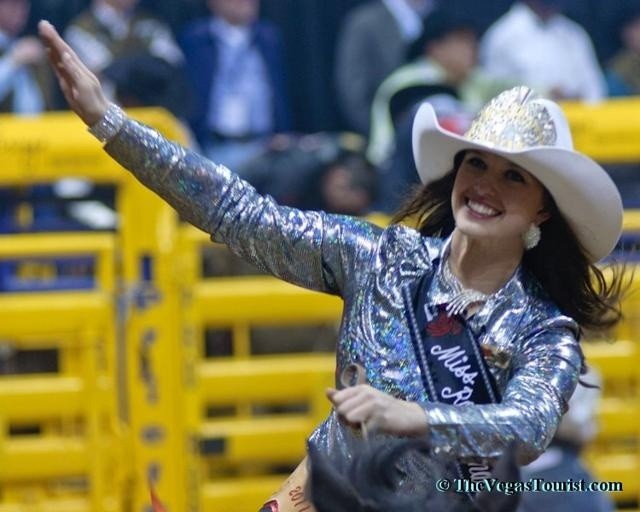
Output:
[411,86,623,263]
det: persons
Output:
[1,0,640,512]
